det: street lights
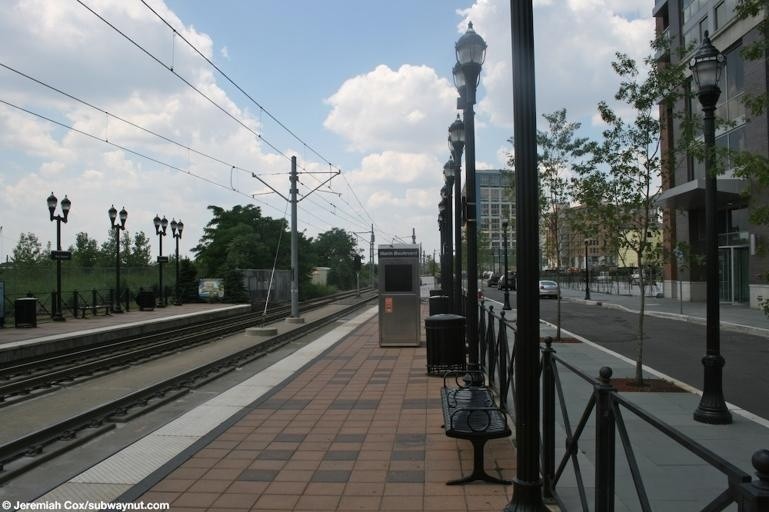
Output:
[688,27,737,427]
[169,217,184,307]
[583,238,593,301]
[433,18,492,388]
[500,218,513,311]
[152,212,169,308]
[46,190,72,323]
[107,203,128,314]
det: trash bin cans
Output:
[139,291,156,311]
[15,297,37,330]
[430,290,444,297]
[424,313,466,378]
[429,295,448,316]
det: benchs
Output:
[78,303,114,320]
[438,369,513,486]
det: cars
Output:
[479,268,561,301]
